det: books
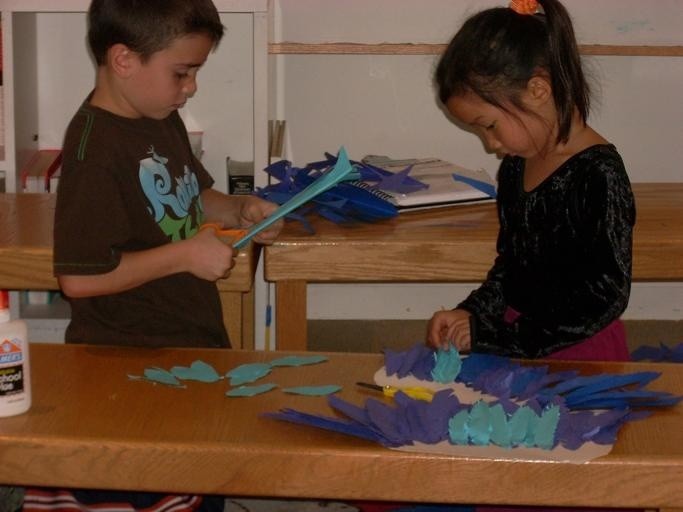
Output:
[349,156,499,213]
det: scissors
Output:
[198,223,256,247]
[356,382,437,403]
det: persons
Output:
[425,0,636,361]
[52,0,286,350]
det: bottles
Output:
[0,292,31,419]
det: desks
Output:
[0,182,683,508]
